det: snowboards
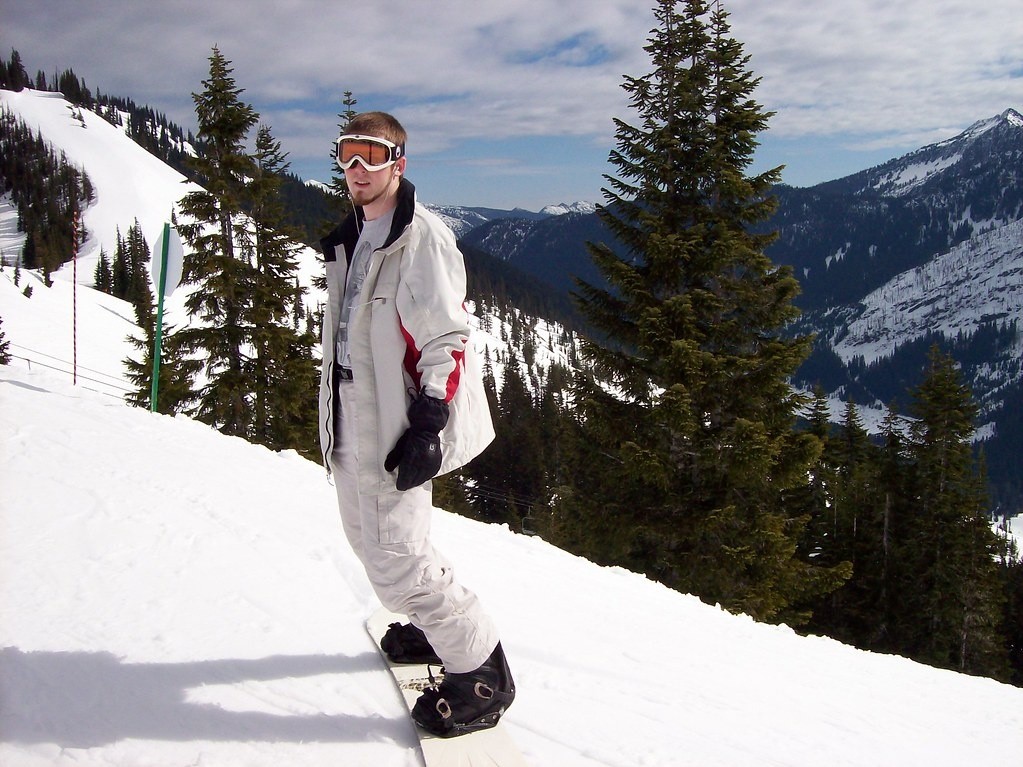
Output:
[364,608,473,767]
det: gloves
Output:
[384,397,449,490]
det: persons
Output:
[318,111,515,738]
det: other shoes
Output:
[381,623,440,666]
[412,642,518,736]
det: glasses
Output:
[332,134,406,171]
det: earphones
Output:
[393,166,400,176]
